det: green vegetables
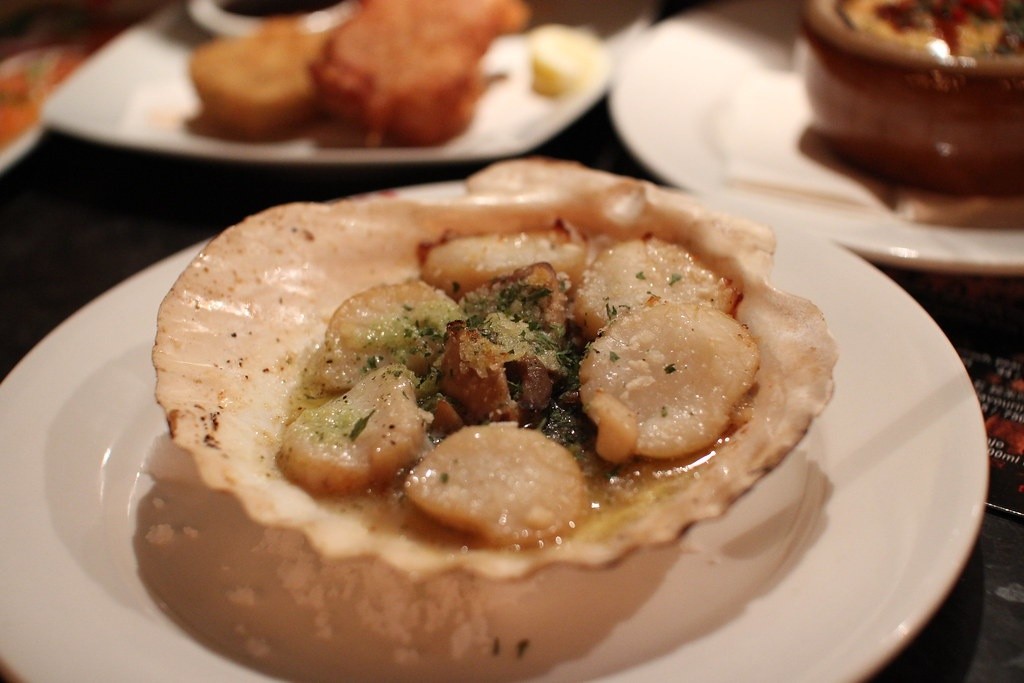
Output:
[349,278,679,442]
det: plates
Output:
[608,0,1024,276]
[35,0,661,162]
[0,182,988,683]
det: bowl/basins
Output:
[802,0,1024,198]
